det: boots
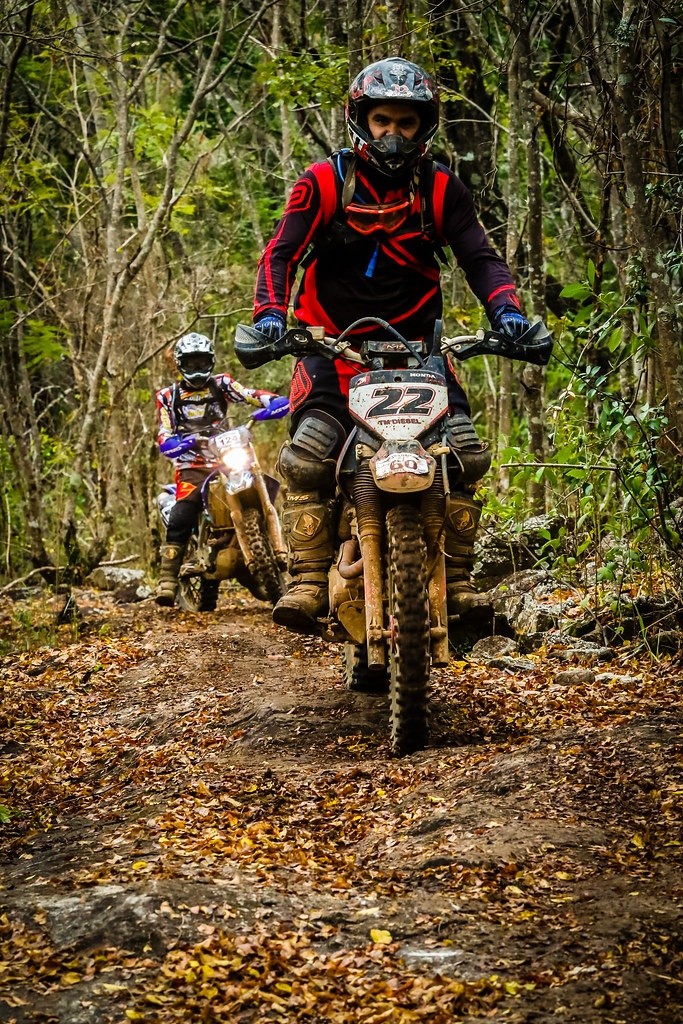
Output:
[272,492,336,633]
[155,544,183,607]
[445,498,493,621]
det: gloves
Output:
[253,312,285,342]
[492,300,529,340]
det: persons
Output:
[246,53,541,637]
[153,333,284,607]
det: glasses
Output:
[344,202,411,235]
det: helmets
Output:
[173,332,216,389]
[344,56,440,181]
